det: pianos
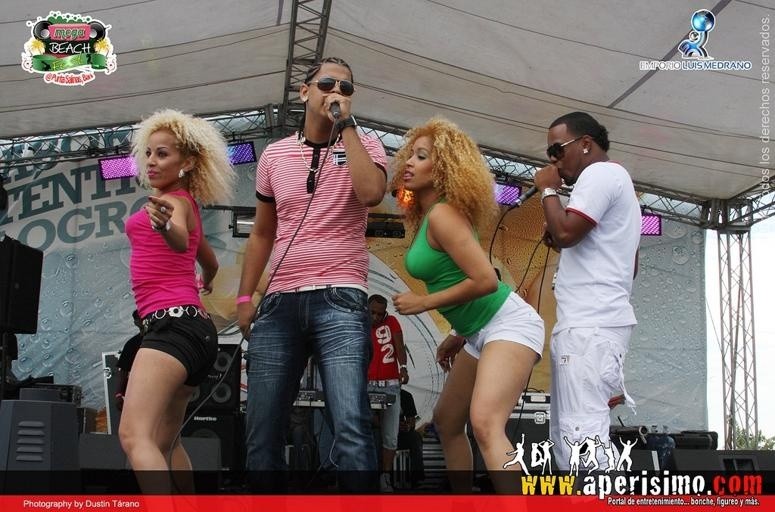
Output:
[292,388,396,411]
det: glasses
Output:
[306,76,355,97]
[546,136,582,159]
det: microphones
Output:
[510,185,538,208]
[330,100,341,118]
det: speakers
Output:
[78,430,221,471]
[667,448,775,472]
[177,344,241,471]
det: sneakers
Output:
[379,472,393,492]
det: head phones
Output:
[384,311,388,319]
